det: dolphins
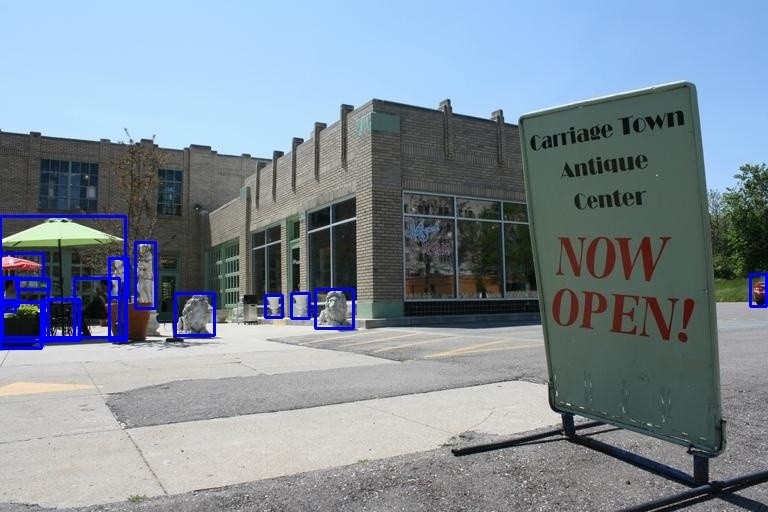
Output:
[176,293,214,336]
[316,289,353,328]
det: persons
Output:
[109,259,129,297]
[135,242,156,308]
[2,278,49,341]
[75,279,120,338]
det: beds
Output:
[16,280,49,289]
[0,217,125,337]
[0,254,43,278]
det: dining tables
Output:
[0,302,42,348]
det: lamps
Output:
[1,301,16,311]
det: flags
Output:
[266,296,282,318]
[291,294,310,318]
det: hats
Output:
[751,275,767,307]
